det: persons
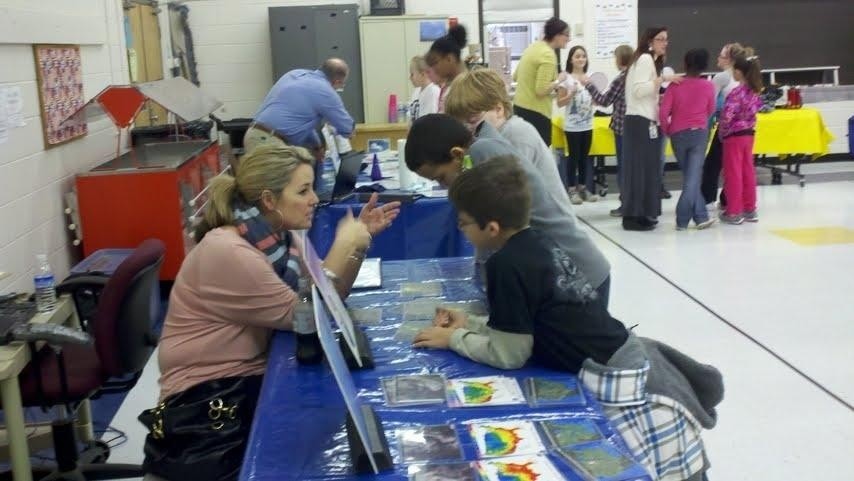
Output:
[411,154,723,481]
[158,143,401,403]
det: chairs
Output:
[2,239,170,481]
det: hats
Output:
[544,15,568,35]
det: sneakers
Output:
[579,184,597,201]
[610,182,759,230]
[568,185,582,204]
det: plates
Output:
[590,71,611,92]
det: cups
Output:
[396,138,414,192]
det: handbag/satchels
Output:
[137,374,254,481]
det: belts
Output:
[248,122,288,143]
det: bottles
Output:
[788,86,795,109]
[33,251,58,313]
[796,88,800,109]
[406,103,410,123]
[397,104,405,123]
[389,93,399,123]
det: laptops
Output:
[317,150,364,203]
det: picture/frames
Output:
[32,41,90,153]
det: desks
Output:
[236,255,655,479]
[549,107,818,197]
[0,291,96,479]
[307,149,468,259]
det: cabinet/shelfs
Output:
[359,14,453,124]
[269,4,365,129]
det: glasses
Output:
[653,35,669,46]
[455,217,474,232]
[336,82,344,91]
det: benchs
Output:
[100,317,175,464]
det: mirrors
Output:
[477,1,563,83]
